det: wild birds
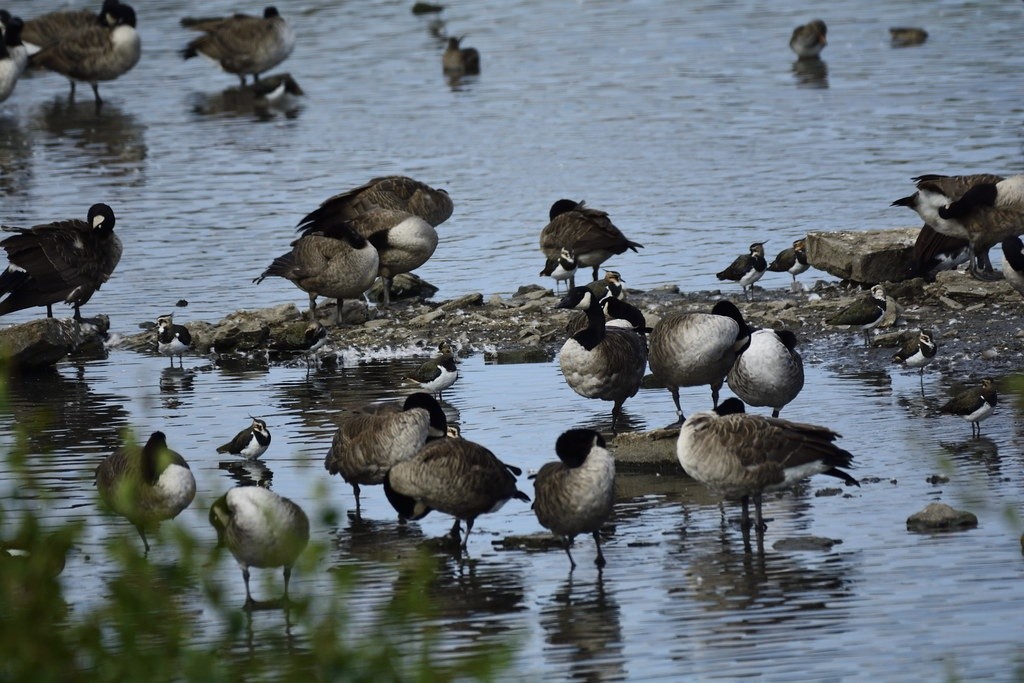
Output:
[824,284,886,344]
[439,34,481,74]
[766,237,810,282]
[153,311,190,363]
[716,239,770,302]
[561,271,626,302]
[215,417,272,460]
[891,327,937,372]
[539,246,578,286]
[788,20,829,59]
[936,378,997,435]
[301,322,327,374]
[402,348,459,398]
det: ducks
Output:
[0,9,28,104]
[175,5,295,86]
[566,297,645,338]
[674,410,861,529]
[538,199,645,281]
[94,431,195,550]
[388,437,531,552]
[252,174,454,334]
[527,426,616,568]
[324,392,448,496]
[384,422,465,525]
[210,484,310,606]
[889,170,1024,283]
[19,0,141,104]
[727,327,805,417]
[652,301,750,430]
[553,286,646,432]
[0,203,123,328]
